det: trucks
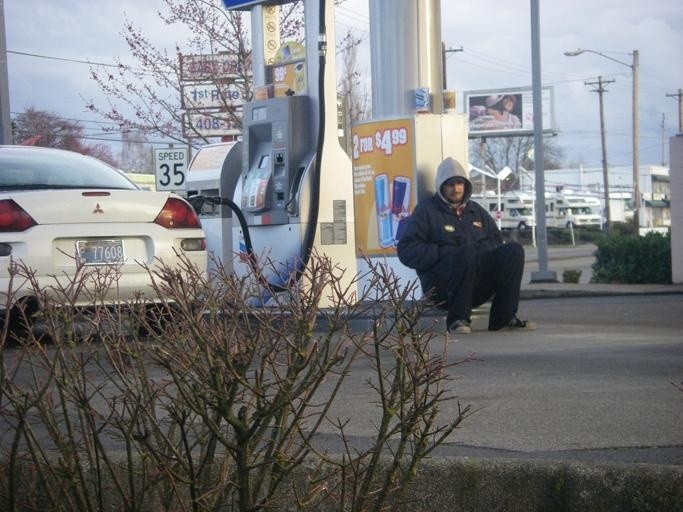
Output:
[471,196,604,230]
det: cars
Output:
[0,143,209,346]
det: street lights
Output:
[563,48,641,207]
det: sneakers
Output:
[448,319,471,333]
[498,314,536,330]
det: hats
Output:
[445,176,467,185]
[486,95,514,106]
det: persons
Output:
[397,156,537,334]
[471,95,522,129]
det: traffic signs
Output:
[154,147,189,191]
